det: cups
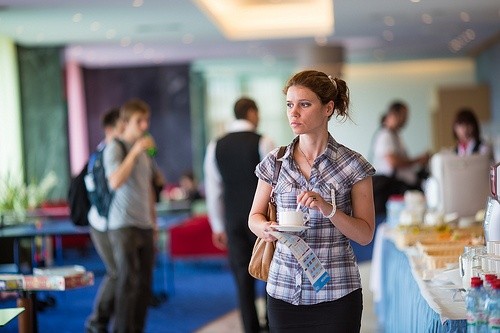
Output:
[278,211,310,226]
[460,241,500,292]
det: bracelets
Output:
[322,189,337,218]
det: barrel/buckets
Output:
[430,156,489,216]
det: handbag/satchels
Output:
[248,147,287,282]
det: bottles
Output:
[466,274,500,333]
[387,195,403,229]
[143,131,157,157]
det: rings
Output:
[313,197,316,201]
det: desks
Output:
[371,220,496,333]
[0,217,186,306]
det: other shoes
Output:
[84,321,110,333]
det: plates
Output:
[272,225,310,233]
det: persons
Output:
[371,99,491,221]
[86,96,276,333]
[248,71,376,333]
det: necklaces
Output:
[296,141,311,167]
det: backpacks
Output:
[70,163,91,225]
[93,139,126,216]
